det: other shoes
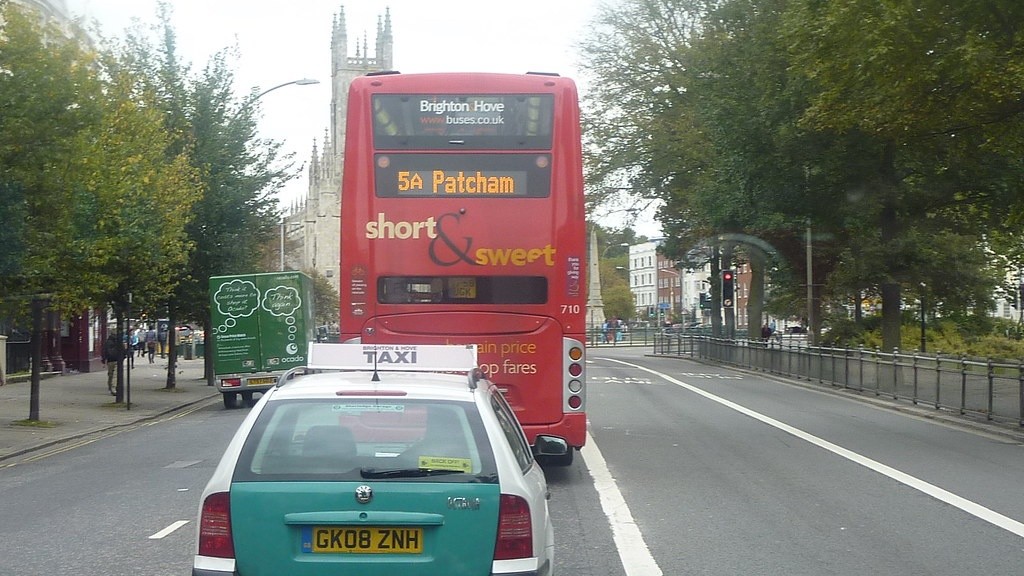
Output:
[112,392,116,396]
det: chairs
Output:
[300,425,356,459]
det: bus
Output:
[338,70,588,466]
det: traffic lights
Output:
[649,306,654,315]
[700,293,706,309]
[721,270,735,308]
[660,308,664,316]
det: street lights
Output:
[602,243,629,260]
[616,266,641,328]
[590,209,637,328]
[280,221,300,270]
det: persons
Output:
[322,320,339,332]
[125,326,180,369]
[602,319,627,344]
[102,329,126,396]
[762,323,771,348]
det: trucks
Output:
[209,271,326,408]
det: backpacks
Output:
[106,339,118,357]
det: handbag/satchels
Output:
[601,333,613,341]
[616,332,622,341]
[148,342,155,348]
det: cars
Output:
[318,324,339,343]
[192,366,569,576]
